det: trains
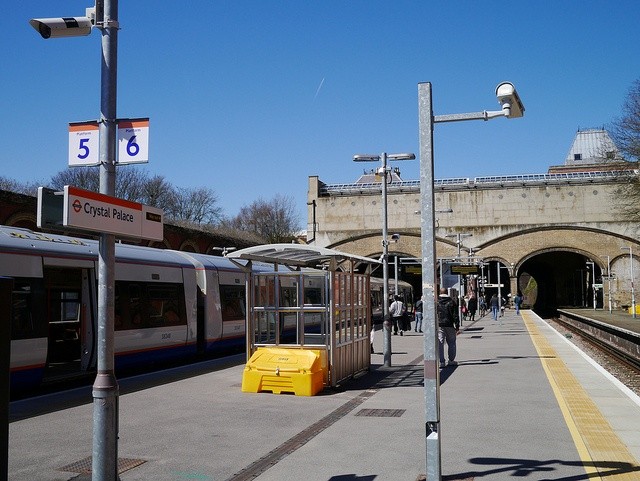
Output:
[0,224,414,370]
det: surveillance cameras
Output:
[392,234,400,239]
[495,81,524,119]
[31,6,97,39]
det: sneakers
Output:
[448,361,457,366]
[440,362,445,367]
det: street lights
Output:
[445,233,473,327]
[618,245,635,320]
[585,261,597,310]
[460,247,481,316]
[473,261,491,296]
[496,262,514,316]
[354,153,417,365]
[598,254,612,315]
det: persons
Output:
[490,293,499,320]
[437,287,460,368]
[388,294,411,336]
[479,294,489,317]
[514,293,522,316]
[414,296,423,334]
[461,294,478,320]
